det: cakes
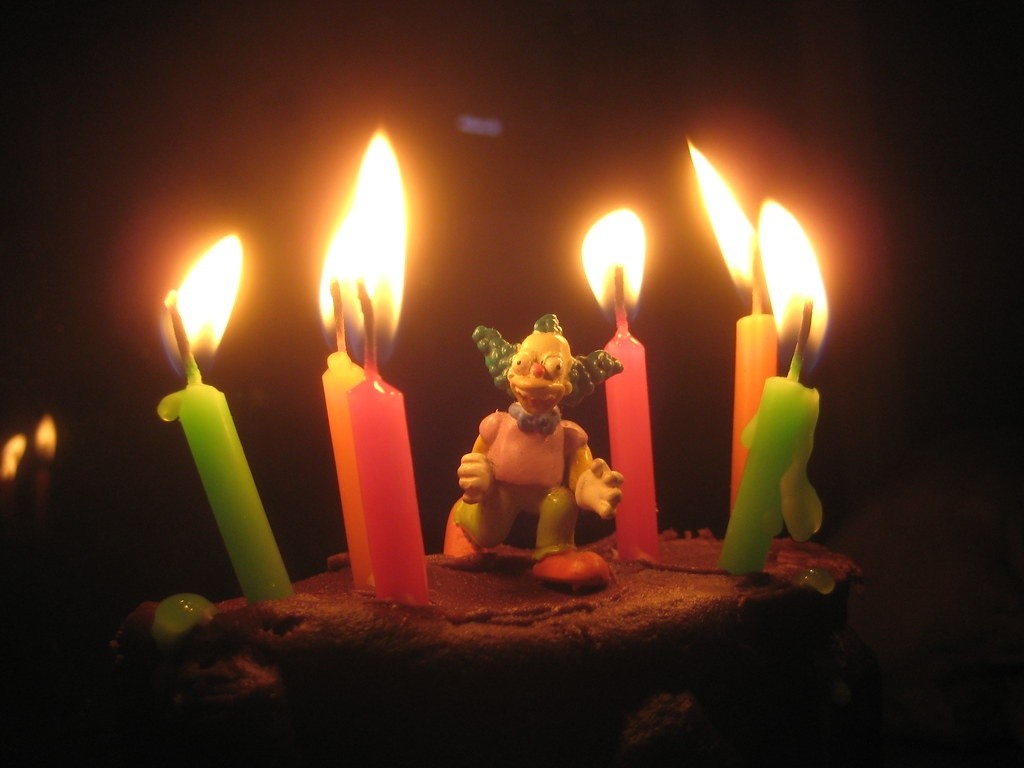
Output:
[122,535,864,766]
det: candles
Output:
[156,230,298,606]
[345,135,438,611]
[578,203,659,567]
[716,196,831,579]
[677,137,781,525]
[320,130,385,595]
